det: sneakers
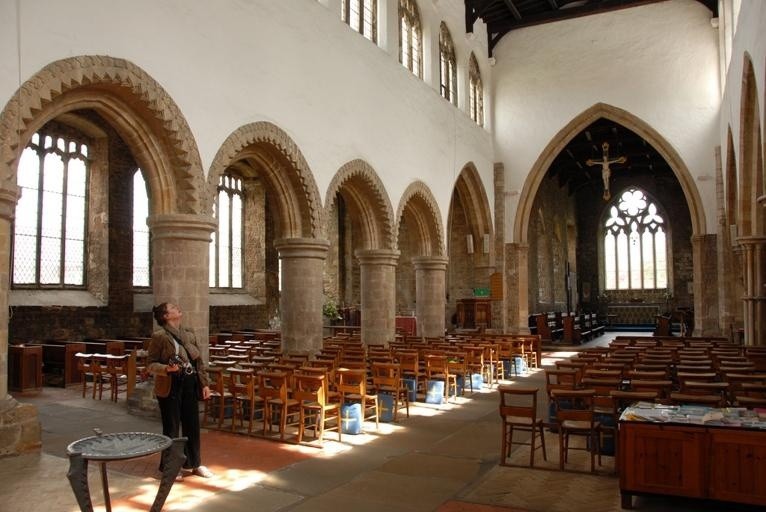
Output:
[175,466,212,481]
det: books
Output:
[618,401,766,429]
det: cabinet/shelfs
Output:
[528,311,672,345]
[616,404,766,509]
[456,298,491,329]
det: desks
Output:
[41,343,86,388]
[8,344,43,393]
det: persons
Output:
[591,155,621,191]
[145,302,214,480]
